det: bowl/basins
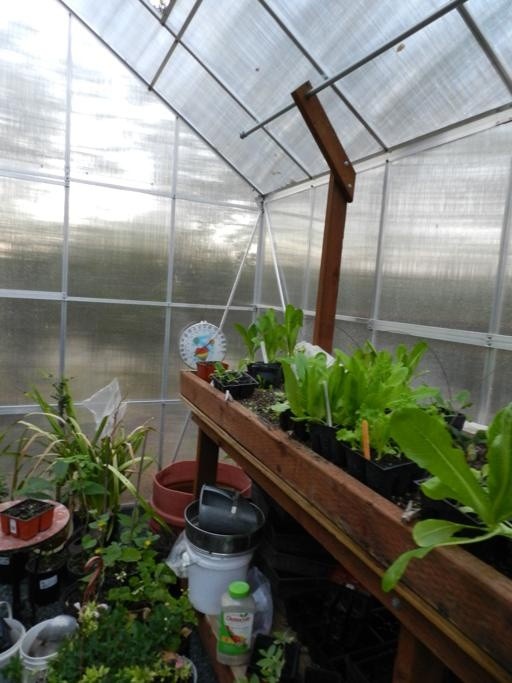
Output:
[183,499,266,554]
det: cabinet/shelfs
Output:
[178,365,512,683]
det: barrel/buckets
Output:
[182,535,256,614]
[0,600,25,668]
[20,615,82,673]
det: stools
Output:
[0,496,71,631]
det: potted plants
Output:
[198,303,512,594]
